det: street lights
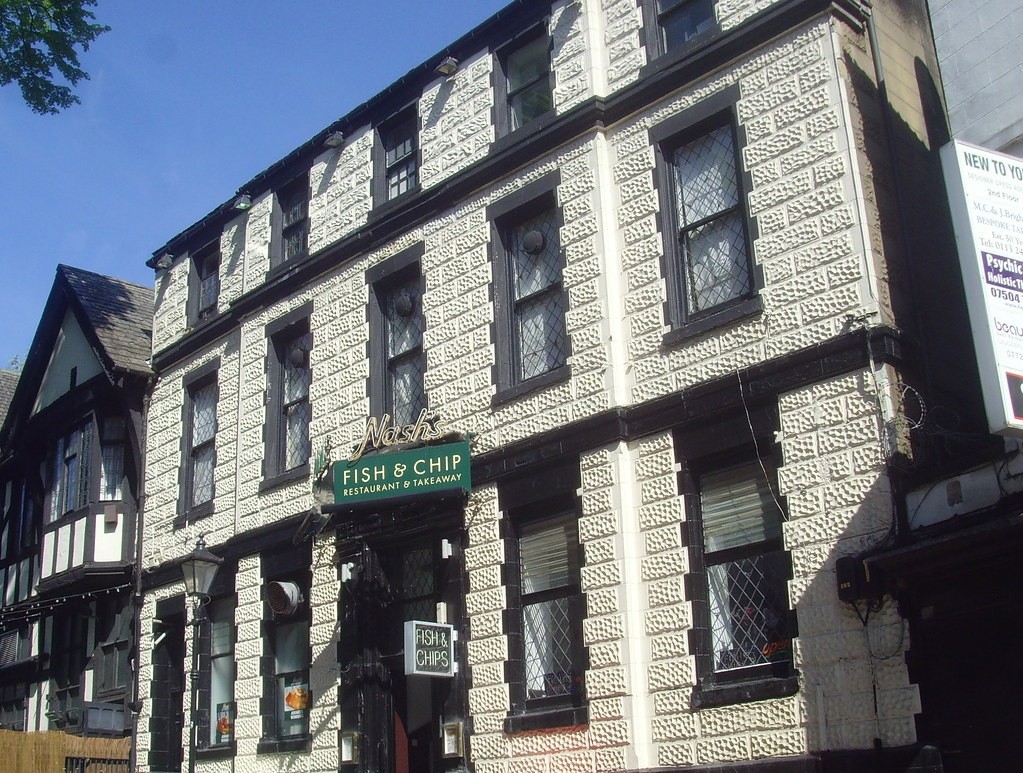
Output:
[176,533,223,773]
